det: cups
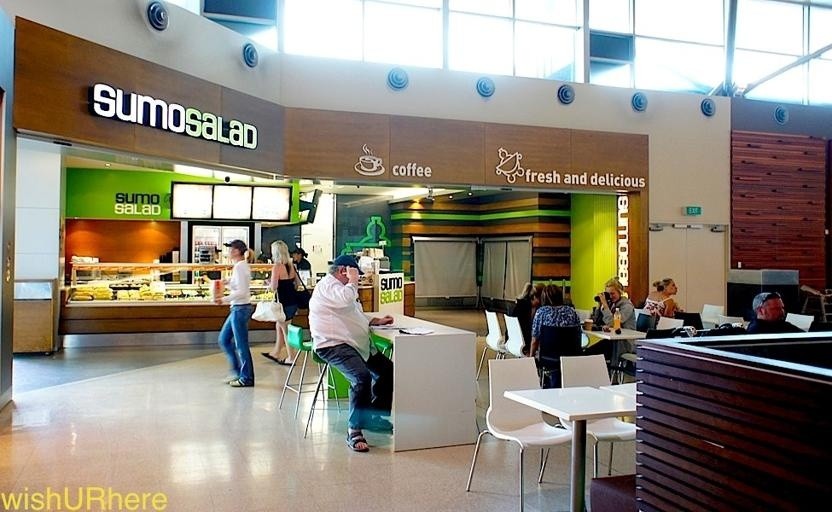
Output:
[584,318,593,331]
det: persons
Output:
[504,282,545,355]
[260,238,299,366]
[205,245,222,279]
[582,275,636,363]
[291,246,313,286]
[744,289,807,334]
[643,277,685,317]
[307,255,395,453]
[527,283,582,388]
[214,240,260,388]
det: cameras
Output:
[594,292,610,303]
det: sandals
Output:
[346,430,369,452]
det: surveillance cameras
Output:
[225,176,230,184]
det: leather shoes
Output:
[276,358,296,366]
[262,352,279,362]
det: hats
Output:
[215,247,222,253]
[222,239,248,254]
[333,255,365,275]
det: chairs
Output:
[278,324,393,439]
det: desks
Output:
[327,312,477,452]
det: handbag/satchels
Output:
[251,302,287,323]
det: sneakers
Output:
[223,376,247,387]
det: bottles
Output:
[613,308,621,330]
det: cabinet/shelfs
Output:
[58,261,311,348]
